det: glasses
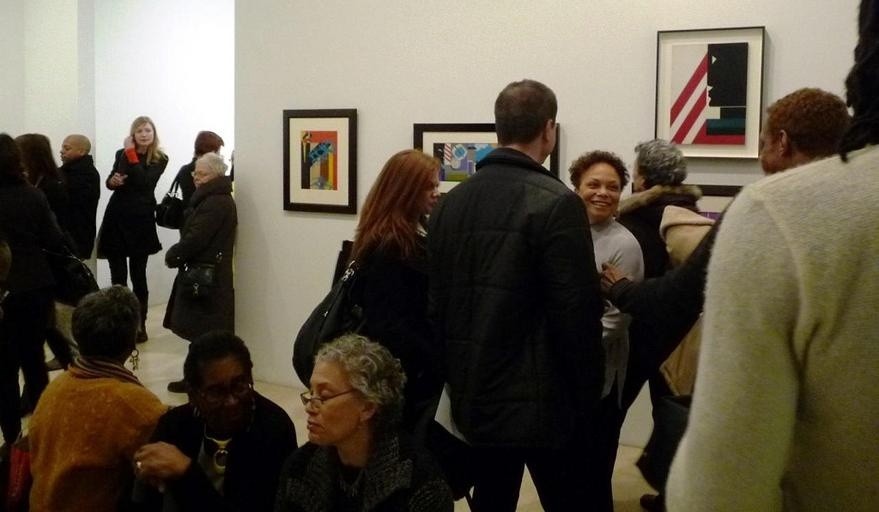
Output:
[300,390,352,409]
[201,379,250,404]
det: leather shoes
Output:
[640,495,666,510]
[46,356,63,369]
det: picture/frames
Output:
[656,25,765,159]
[413,122,560,198]
[630,181,743,219]
[282,108,358,215]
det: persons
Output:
[349,80,716,511]
[1,116,238,441]
[664,1,879,512]
[29,281,455,512]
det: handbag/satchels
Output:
[6,437,30,509]
[48,254,98,305]
[156,194,185,228]
[293,267,360,386]
[180,263,217,298]
